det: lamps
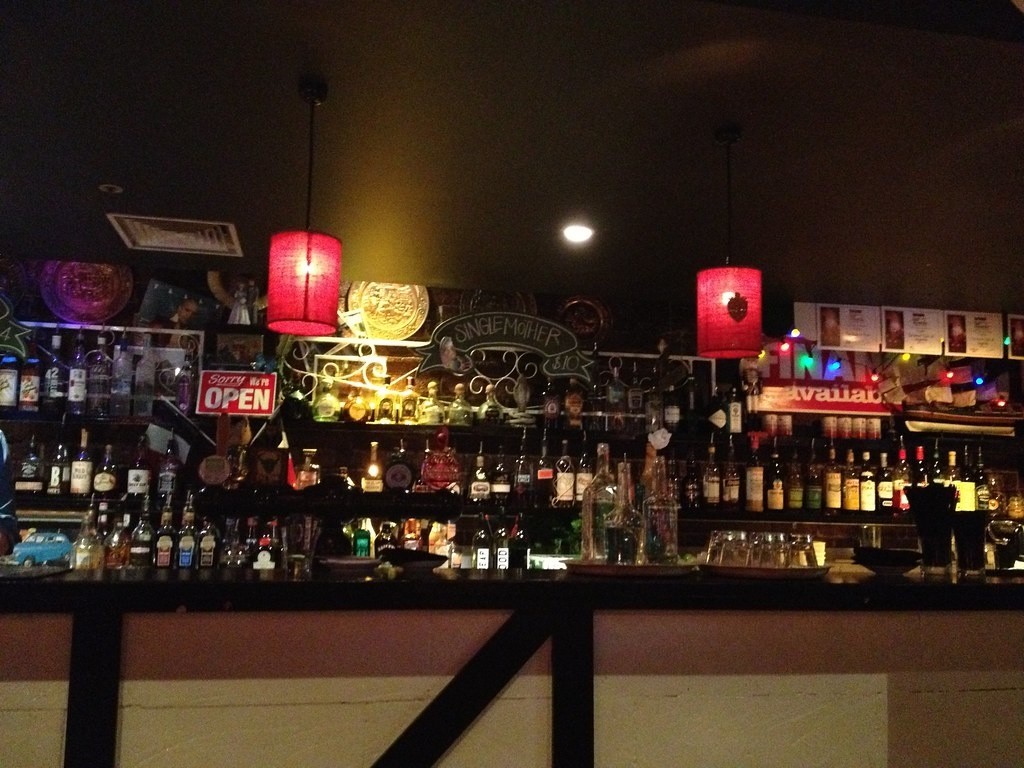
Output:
[265,74,343,337]
[692,124,763,359]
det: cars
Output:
[12,532,73,568]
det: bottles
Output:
[0,332,1024,571]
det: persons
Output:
[146,297,199,348]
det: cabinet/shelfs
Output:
[273,423,919,529]
[0,411,199,508]
